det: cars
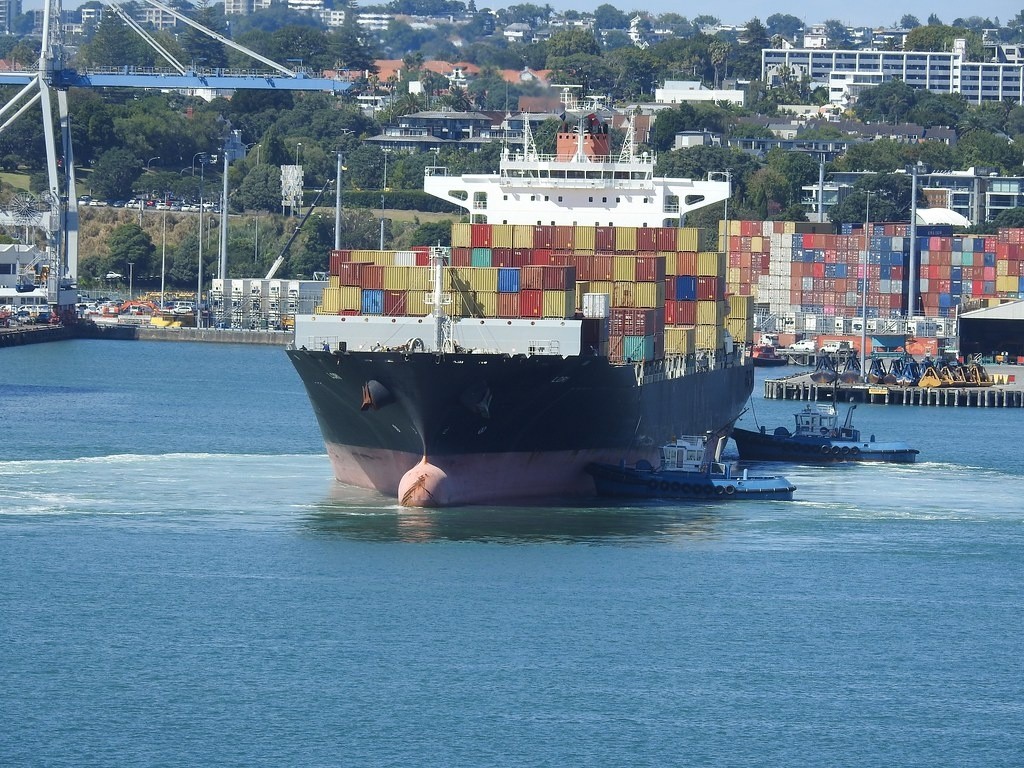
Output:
[0,299,116,322]
[105,271,123,279]
[78,192,221,214]
[789,339,819,353]
[173,305,192,315]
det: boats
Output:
[594,413,797,503]
[284,106,756,508]
[866,353,994,389]
[730,375,920,464]
[838,348,863,384]
[809,350,840,384]
[750,343,789,366]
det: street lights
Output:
[147,156,160,169]
[296,143,302,165]
[192,152,206,328]
[256,144,262,164]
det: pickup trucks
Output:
[820,341,860,355]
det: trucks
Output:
[757,333,784,351]
